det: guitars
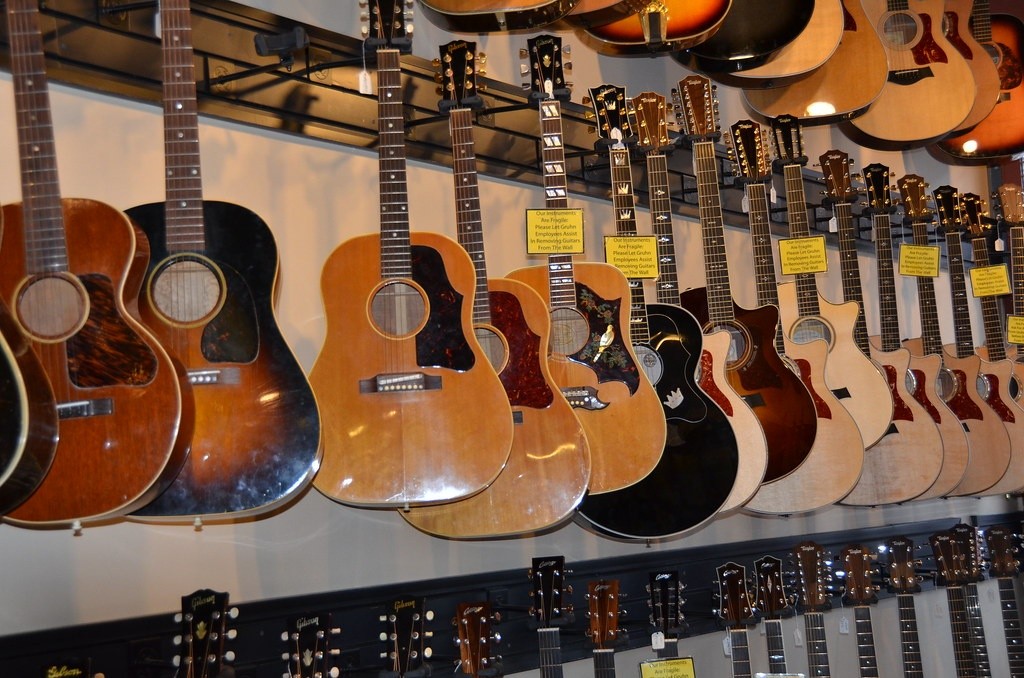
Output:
[397,39,593,541]
[624,92,769,518]
[169,521,1024,678]
[503,33,669,498]
[767,113,894,451]
[308,1,515,510]
[0,0,195,531]
[723,118,867,519]
[570,83,739,543]
[119,2,324,527]
[854,162,971,502]
[417,0,1024,168]
[893,172,1024,498]
[812,149,946,508]
[678,69,819,489]
